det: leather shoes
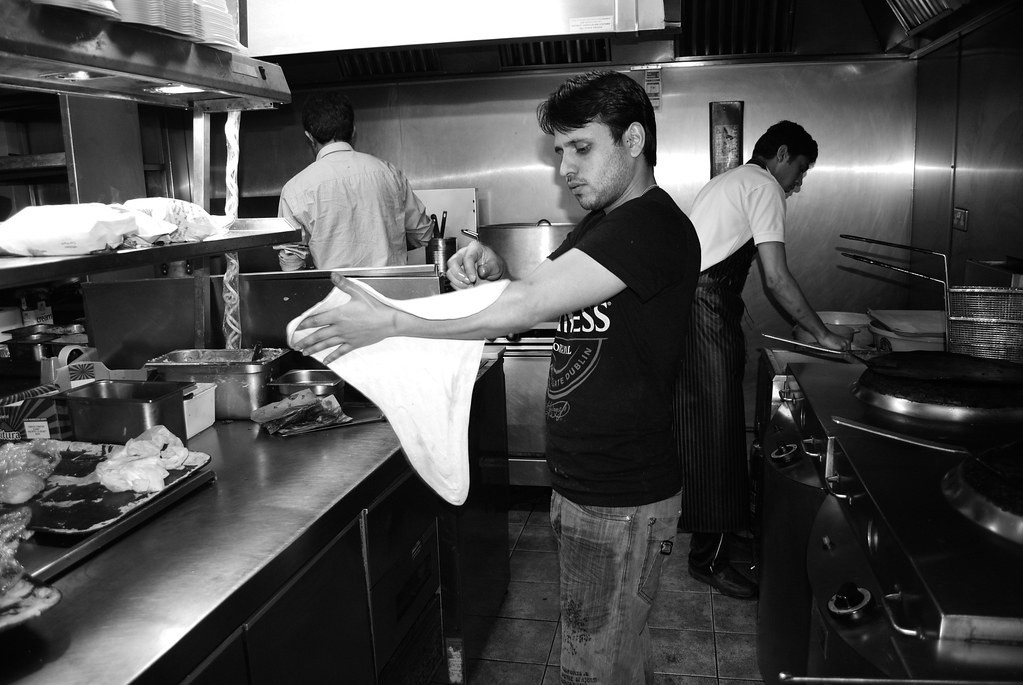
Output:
[688,564,757,597]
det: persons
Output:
[293,70,700,685]
[274,91,433,272]
[682,116,859,600]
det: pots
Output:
[760,331,1022,424]
[830,416,1023,544]
[478,222,579,281]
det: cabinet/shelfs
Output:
[187,373,513,685]
